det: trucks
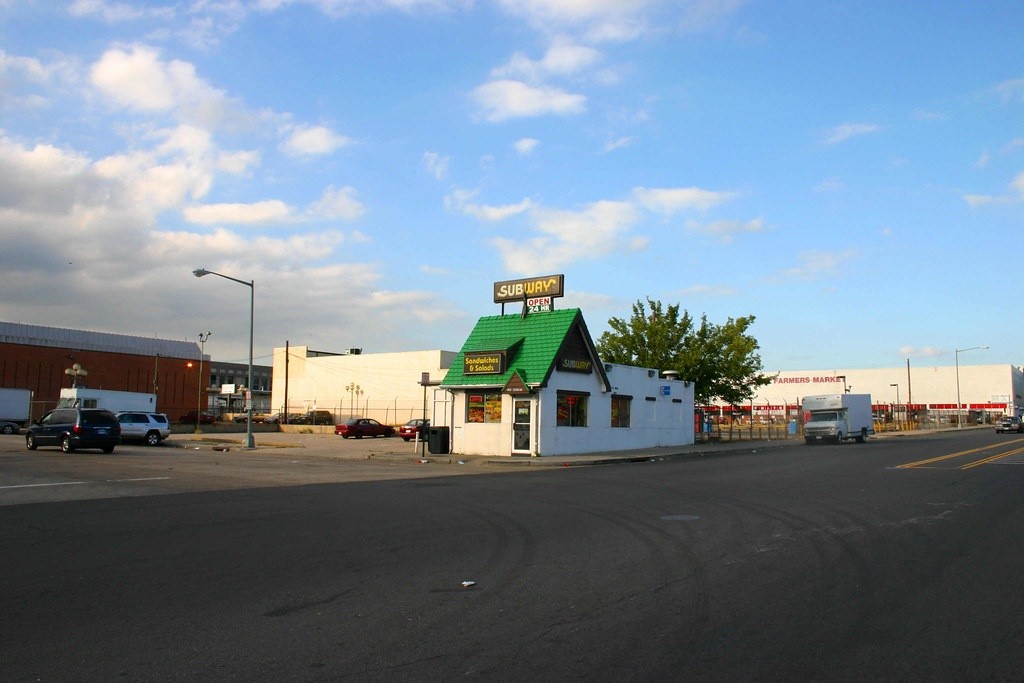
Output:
[55,387,157,417]
[799,393,874,445]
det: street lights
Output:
[890,383,901,431]
[192,268,256,449]
[955,345,989,430]
[193,331,211,434]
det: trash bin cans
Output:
[790,421,798,434]
[428,426,449,454]
[703,422,712,432]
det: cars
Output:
[994,416,1024,434]
[179,410,215,425]
[334,418,395,439]
[263,410,304,424]
[234,411,268,424]
[399,418,430,441]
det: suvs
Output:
[295,410,333,425]
[25,406,122,453]
[92,410,171,446]
